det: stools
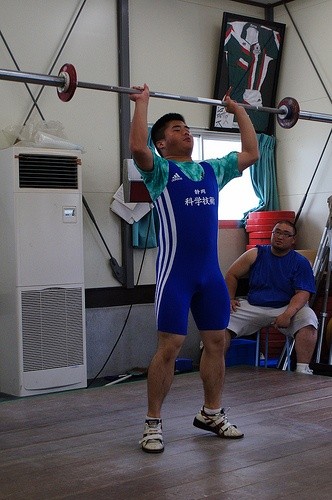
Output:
[255,330,290,372]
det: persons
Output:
[128,83,258,452]
[224,220,317,374]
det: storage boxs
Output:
[225,339,257,367]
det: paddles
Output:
[103,367,147,387]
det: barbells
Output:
[0,63,332,129]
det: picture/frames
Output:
[210,12,286,135]
